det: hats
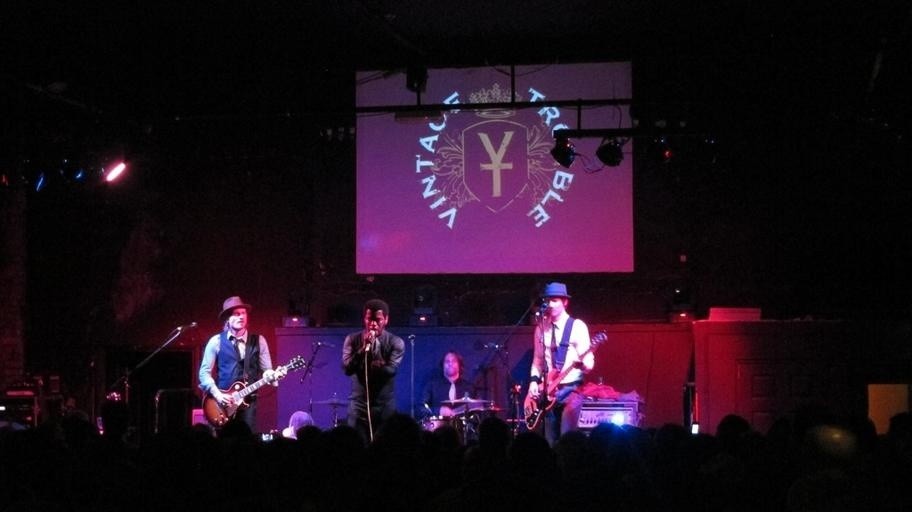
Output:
[218,296,252,321]
[543,283,571,298]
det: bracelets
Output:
[529,375,542,384]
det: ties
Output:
[233,337,244,355]
[550,322,559,368]
[449,382,456,401]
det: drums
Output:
[455,408,482,446]
[424,416,453,443]
[507,420,526,436]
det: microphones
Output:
[176,321,198,331]
[313,341,336,349]
[535,302,548,317]
[364,330,376,352]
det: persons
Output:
[422,350,483,418]
[343,299,405,429]
[523,282,596,448]
[0,394,912,512]
[198,295,288,433]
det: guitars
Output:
[523,332,608,431]
[203,355,306,428]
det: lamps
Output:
[553,103,721,170]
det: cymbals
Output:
[485,406,504,411]
[440,397,491,404]
